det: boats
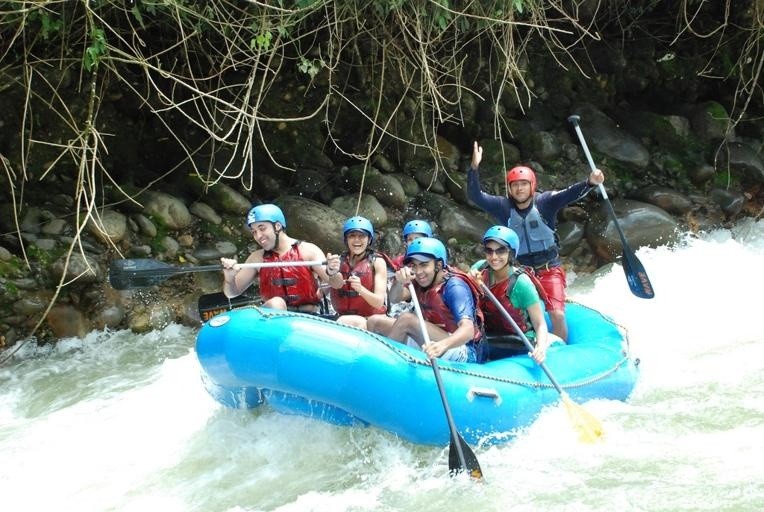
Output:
[195,300,640,447]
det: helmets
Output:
[247,204,286,229]
[507,166,536,198]
[342,215,373,246]
[483,225,520,259]
[407,237,446,268]
[403,220,432,240]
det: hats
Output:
[408,254,432,263]
[346,229,368,235]
[485,237,508,248]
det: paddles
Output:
[406,271,485,480]
[109,258,328,291]
[197,279,350,324]
[568,115,655,300]
[475,279,602,444]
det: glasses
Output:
[485,247,505,254]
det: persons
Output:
[374,237,488,364]
[393,219,433,270]
[220,203,344,314]
[468,224,550,367]
[317,215,398,331]
[466,140,605,342]
